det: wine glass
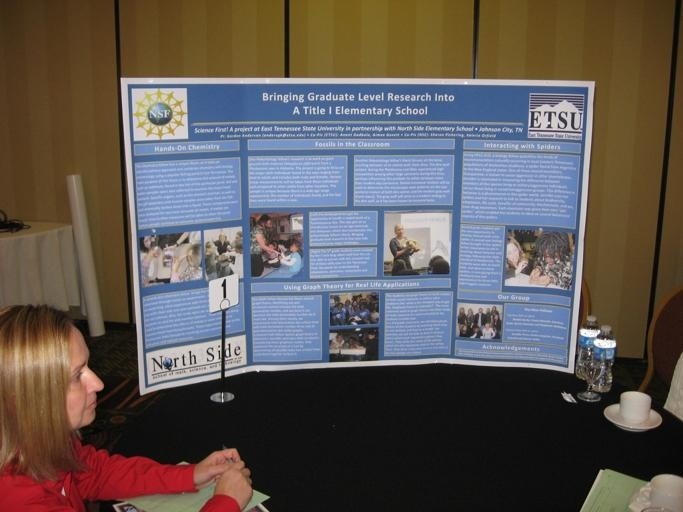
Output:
[575,344,605,402]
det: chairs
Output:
[580,277,590,329]
[637,285,683,407]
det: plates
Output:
[602,403,661,433]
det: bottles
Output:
[591,324,616,394]
[574,315,601,380]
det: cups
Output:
[635,472,682,512]
[619,390,652,426]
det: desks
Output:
[0,221,81,312]
[98,364,683,512]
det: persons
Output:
[137,232,202,285]
[330,329,379,361]
[1,303,252,511]
[458,305,502,341]
[389,224,449,275]
[249,212,305,281]
[530,230,574,290]
[330,293,380,324]
[203,226,246,281]
[504,237,533,280]
